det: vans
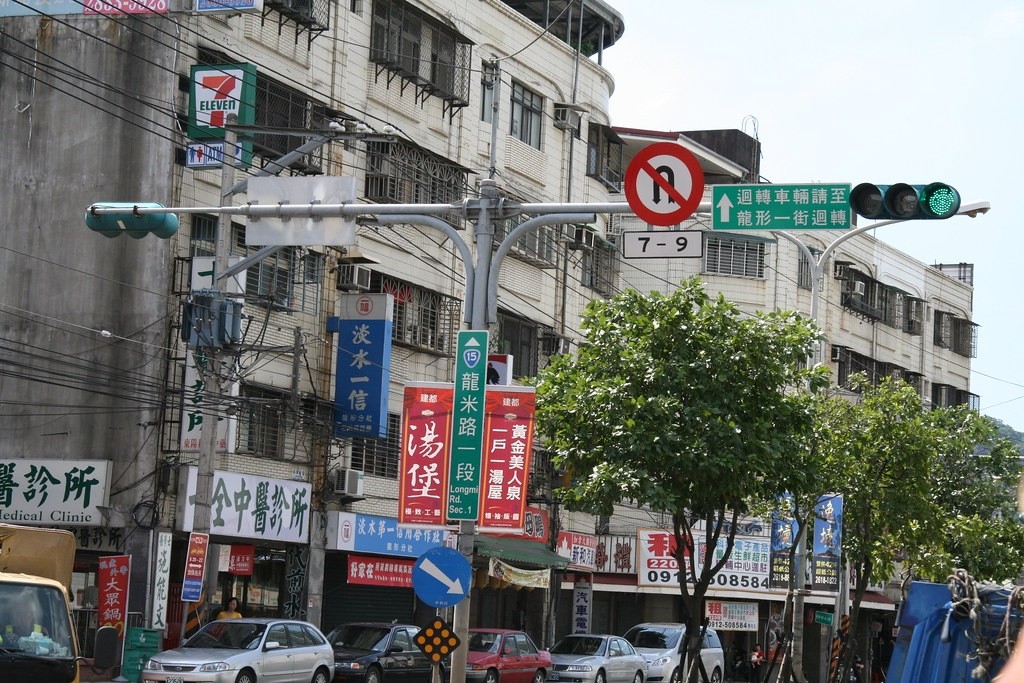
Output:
[623,623,724,683]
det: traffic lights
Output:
[845,181,961,220]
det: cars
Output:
[140,618,333,683]
[466,629,553,683]
[326,622,451,683]
[544,634,648,683]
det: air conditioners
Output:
[337,263,371,290]
[335,467,364,496]
[848,281,865,295]
[570,228,594,250]
[553,108,578,131]
[832,347,846,361]
[561,224,575,243]
[835,264,849,279]
[415,326,431,348]
[367,155,401,180]
[543,336,569,356]
[907,320,921,335]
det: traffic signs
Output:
[711,185,855,228]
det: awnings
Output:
[474,535,571,569]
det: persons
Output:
[216,597,242,621]
[750,644,765,683]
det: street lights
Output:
[683,200,993,683]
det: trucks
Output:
[0,522,122,683]
